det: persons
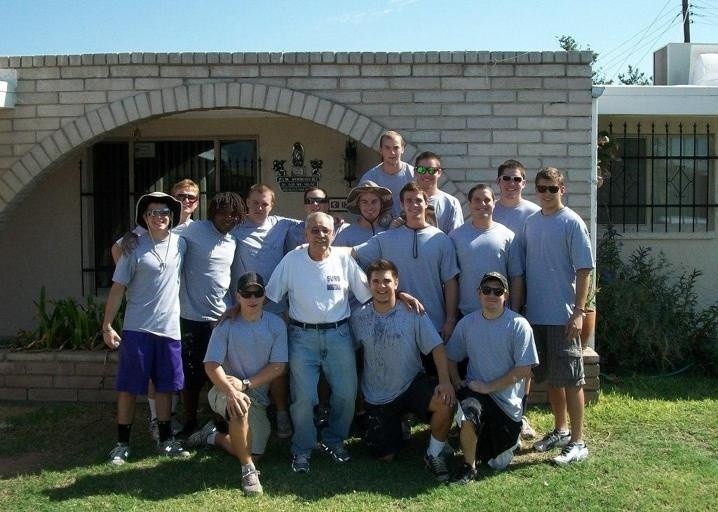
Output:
[102,179,200,464]
[363,132,463,233]
[445,162,594,487]
[188,182,463,494]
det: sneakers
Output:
[522,415,571,451]
[150,414,217,456]
[109,442,129,465]
[445,463,484,485]
[553,441,588,464]
[423,450,449,481]
[234,413,350,496]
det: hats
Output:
[238,272,265,291]
[480,272,508,292]
[347,180,393,214]
[134,191,182,229]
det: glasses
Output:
[304,197,326,204]
[481,286,503,296]
[536,186,560,193]
[499,175,524,182]
[238,289,263,298]
[310,228,332,233]
[415,165,440,174]
[174,193,198,203]
[145,208,171,217]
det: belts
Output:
[289,318,347,329]
